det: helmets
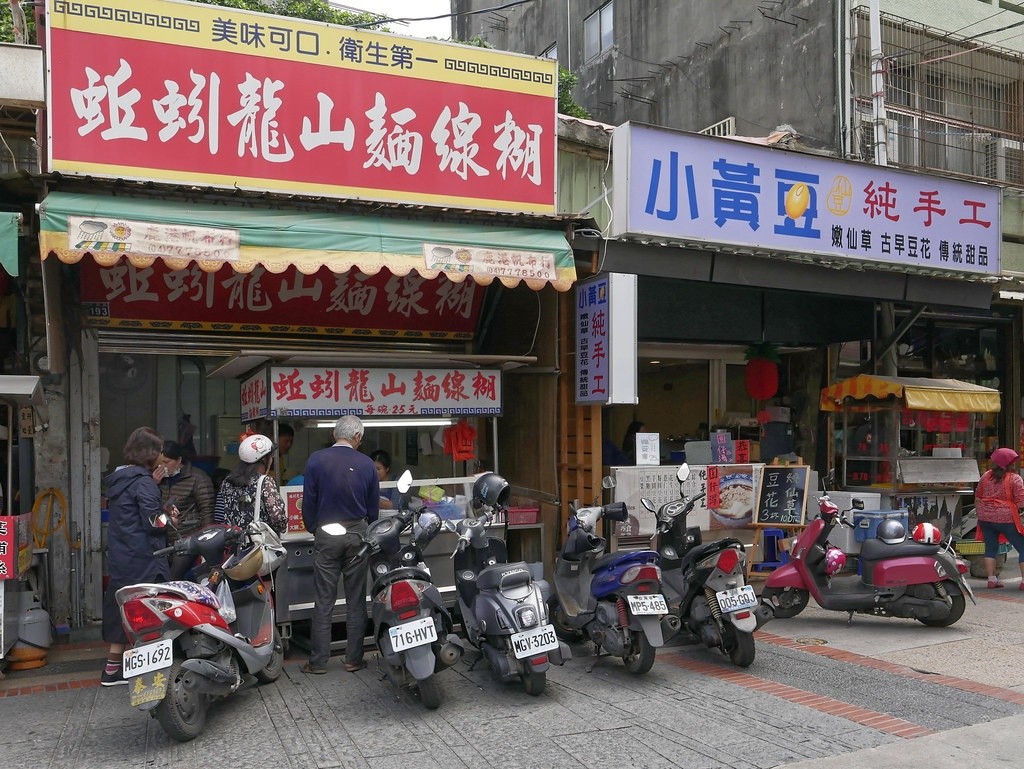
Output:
[912,522,941,545]
[414,510,441,550]
[877,517,906,545]
[473,473,510,512]
[222,542,263,582]
[825,547,846,576]
[238,434,279,464]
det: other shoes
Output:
[988,580,1004,588]
[301,662,327,674]
[1020,583,1024,590]
[345,659,367,671]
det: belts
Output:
[317,519,360,527]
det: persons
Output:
[152,439,216,580]
[100,426,180,686]
[602,420,647,466]
[298,415,379,674]
[975,448,1024,591]
[214,422,295,627]
[370,449,405,510]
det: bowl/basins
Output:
[710,471,752,527]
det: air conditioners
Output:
[980,138,1024,188]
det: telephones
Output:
[753,465,811,525]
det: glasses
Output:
[269,453,276,458]
[356,432,362,445]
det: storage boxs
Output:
[826,524,862,554]
[853,508,909,543]
[807,490,881,523]
[765,406,791,423]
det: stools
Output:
[754,527,787,572]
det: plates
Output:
[295,498,303,511]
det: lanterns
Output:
[745,358,778,401]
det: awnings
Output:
[0,211,24,278]
[39,188,578,292]
[820,374,1001,412]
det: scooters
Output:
[544,476,682,676]
[640,461,776,669]
[114,493,285,742]
[320,469,466,711]
[760,468,977,627]
[443,503,575,698]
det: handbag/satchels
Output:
[245,475,287,576]
[215,579,236,625]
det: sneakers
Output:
[101,668,129,686]
